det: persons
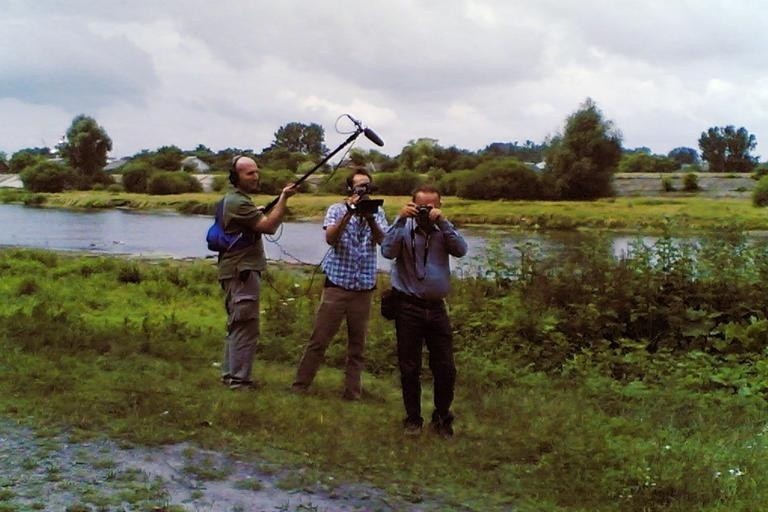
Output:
[291,169,389,400]
[382,185,470,438]
[221,154,294,390]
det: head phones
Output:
[229,155,244,186]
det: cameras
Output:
[415,203,434,221]
[346,182,384,217]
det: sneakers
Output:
[437,415,453,439]
[404,414,424,439]
[344,388,361,401]
[291,381,309,397]
[220,371,261,392]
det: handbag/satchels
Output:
[381,289,396,320]
[206,222,245,252]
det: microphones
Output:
[363,127,384,147]
